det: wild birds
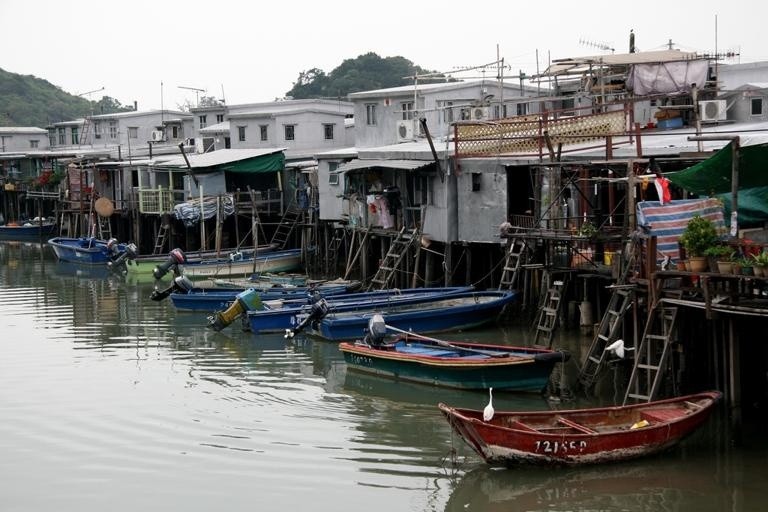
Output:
[482,386,495,422]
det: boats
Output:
[339,316,572,396]
[48,237,137,265]
[438,390,723,469]
[0,217,58,235]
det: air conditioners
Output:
[396,120,419,142]
[471,107,493,120]
[152,131,162,141]
[698,100,727,122]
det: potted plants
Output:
[677,213,768,276]
